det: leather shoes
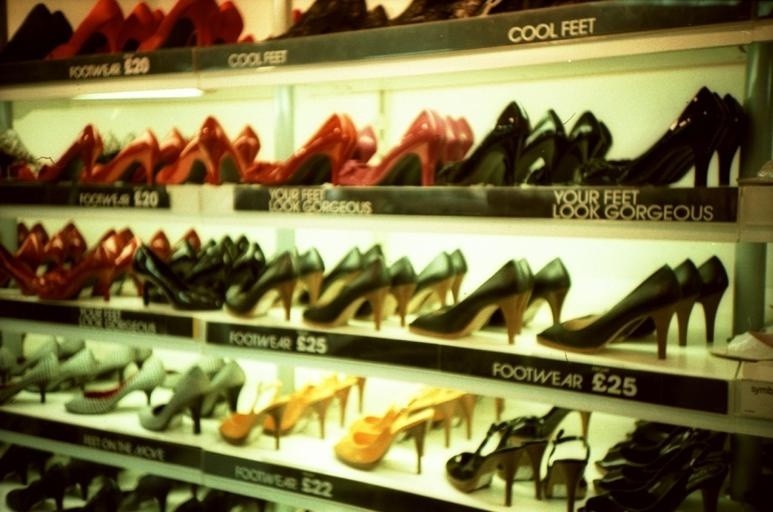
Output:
[0,3,73,63]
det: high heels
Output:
[387,1,509,28]
[537,264,679,360]
[697,255,729,343]
[667,92,732,189]
[182,360,245,419]
[611,258,703,346]
[403,389,477,439]
[710,94,750,187]
[350,393,459,448]
[35,124,102,184]
[125,127,185,184]
[242,112,353,186]
[262,380,336,439]
[134,0,254,52]
[26,347,97,391]
[65,359,166,415]
[155,116,221,186]
[383,113,474,187]
[577,86,726,189]
[577,122,611,184]
[354,256,418,327]
[199,116,245,185]
[418,248,468,314]
[225,248,325,322]
[132,245,222,311]
[300,371,354,428]
[495,415,550,500]
[59,474,198,512]
[86,343,132,383]
[408,258,528,345]
[218,381,290,451]
[487,257,570,327]
[163,357,226,403]
[127,344,152,371]
[264,0,389,40]
[138,365,211,434]
[435,101,530,186]
[327,374,366,413]
[336,110,436,188]
[220,125,261,186]
[395,251,456,315]
[537,109,601,184]
[514,109,566,186]
[302,116,377,185]
[511,407,592,447]
[0,443,52,486]
[541,429,590,512]
[335,400,434,474]
[1,352,59,407]
[479,259,534,335]
[81,126,159,184]
[303,262,393,331]
[297,244,386,319]
[577,419,731,512]
[0,222,202,299]
[168,484,273,512]
[44,0,164,60]
[7,458,126,512]
[445,417,537,506]
[143,234,265,307]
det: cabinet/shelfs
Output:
[734,0,773,439]
[0,48,202,512]
[194,0,759,512]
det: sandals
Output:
[709,329,773,363]
